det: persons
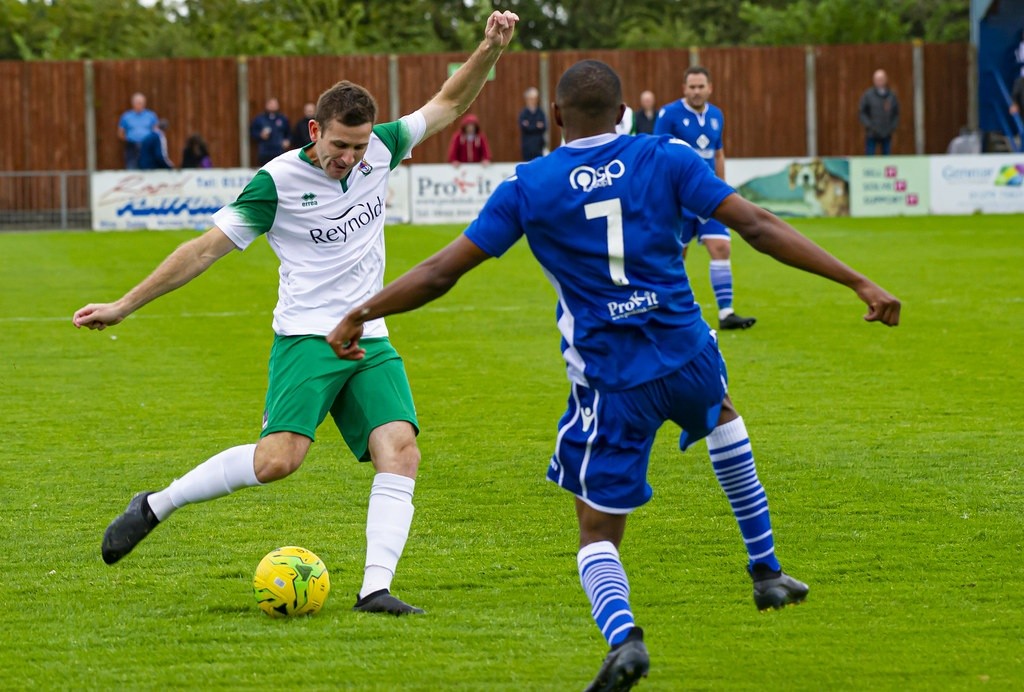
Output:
[652,68,756,329]
[615,91,658,134]
[325,59,902,692]
[1009,77,1024,117]
[859,69,899,155]
[518,87,547,162]
[448,114,491,165]
[72,10,521,614]
[183,134,212,167]
[250,98,316,166]
[118,92,174,168]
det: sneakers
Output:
[101,490,161,564]
[583,635,652,692]
[752,574,809,614]
[718,312,756,330]
[352,588,426,617]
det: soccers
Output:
[252,545,331,618]
